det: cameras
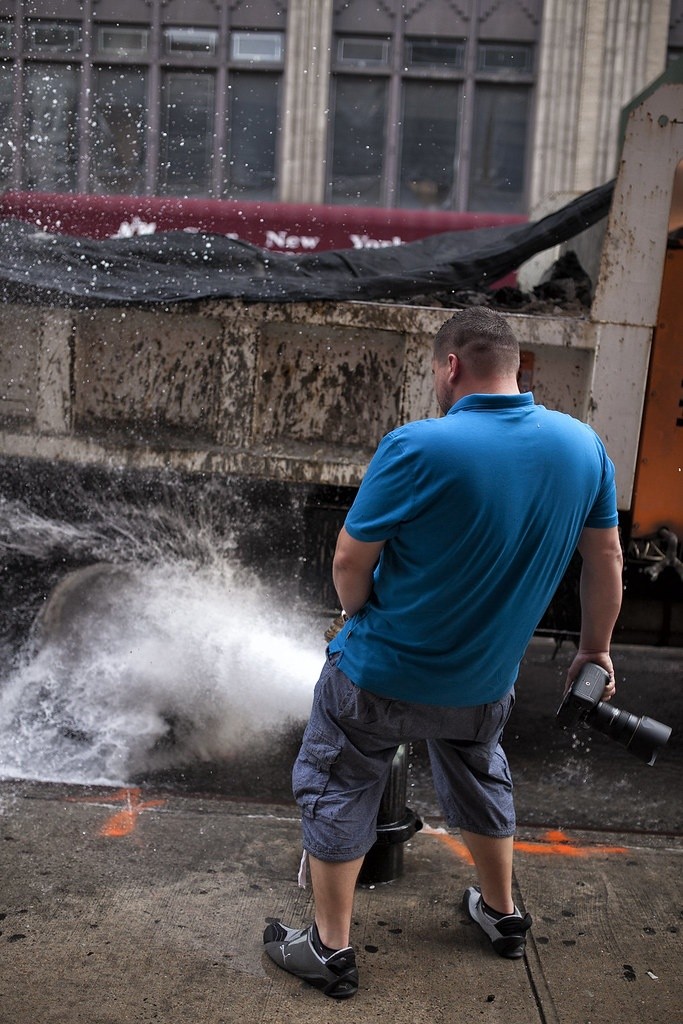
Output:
[555,661,672,769]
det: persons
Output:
[254,306,629,998]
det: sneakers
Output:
[463,884,531,960]
[262,923,359,998]
